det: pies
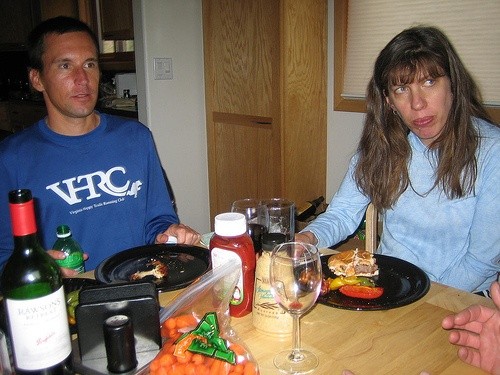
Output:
[327,248,379,277]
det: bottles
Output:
[209,212,256,318]
[0,188,78,375]
[296,196,324,220]
[51,225,87,334]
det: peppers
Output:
[323,277,383,298]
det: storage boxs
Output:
[295,204,383,250]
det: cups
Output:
[230,197,296,265]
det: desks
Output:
[80,248,496,375]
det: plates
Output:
[93,243,213,292]
[293,253,430,312]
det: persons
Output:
[0,16,201,277]
[442,281,500,375]
[291,26,500,293]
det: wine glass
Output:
[268,241,323,375]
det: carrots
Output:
[145,313,259,375]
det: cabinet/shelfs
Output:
[0,0,134,62]
